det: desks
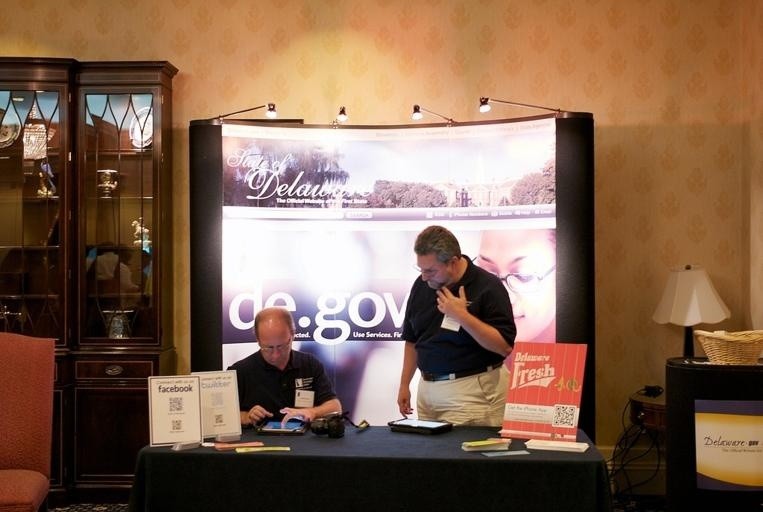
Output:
[629,392,666,433]
[140,424,605,511]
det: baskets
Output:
[694,329,763,365]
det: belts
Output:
[417,362,501,381]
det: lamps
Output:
[412,104,458,124]
[332,105,348,129]
[651,265,731,358]
[479,96,568,113]
[211,103,278,119]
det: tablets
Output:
[387,417,453,436]
[256,417,311,436]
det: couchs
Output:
[0,331,55,512]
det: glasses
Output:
[259,338,293,353]
[413,265,446,277]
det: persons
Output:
[227,308,342,428]
[478,228,556,372]
[85,240,139,293]
[397,226,517,427]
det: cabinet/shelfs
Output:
[665,355,762,511]
[50,354,68,508]
[68,354,159,505]
[79,61,180,354]
[0,57,79,354]
